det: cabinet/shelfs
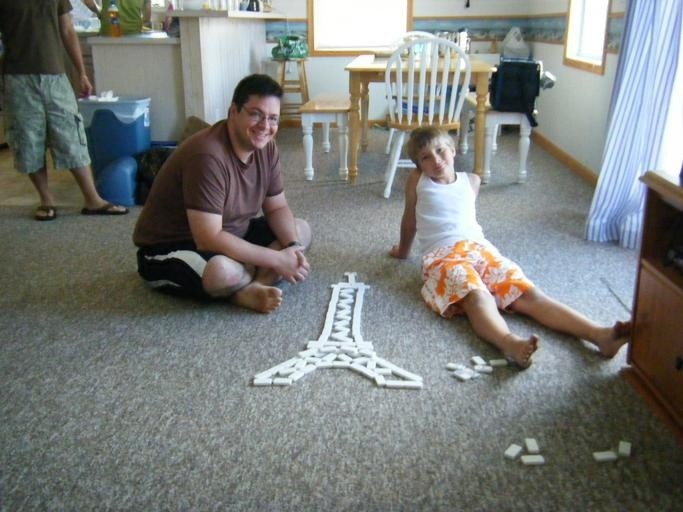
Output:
[623,167,683,443]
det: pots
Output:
[431,30,471,58]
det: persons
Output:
[82,0,151,36]
[389,126,631,369]
[0,0,129,221]
[133,74,312,315]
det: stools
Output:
[297,92,351,181]
[272,58,309,132]
[458,91,538,186]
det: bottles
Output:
[106,1,120,38]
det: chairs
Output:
[384,31,441,155]
[382,36,471,199]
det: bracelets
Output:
[285,241,302,248]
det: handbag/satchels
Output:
[272,35,308,58]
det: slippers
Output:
[35,206,55,220]
[81,201,128,215]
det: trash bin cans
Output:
[80,94,151,205]
